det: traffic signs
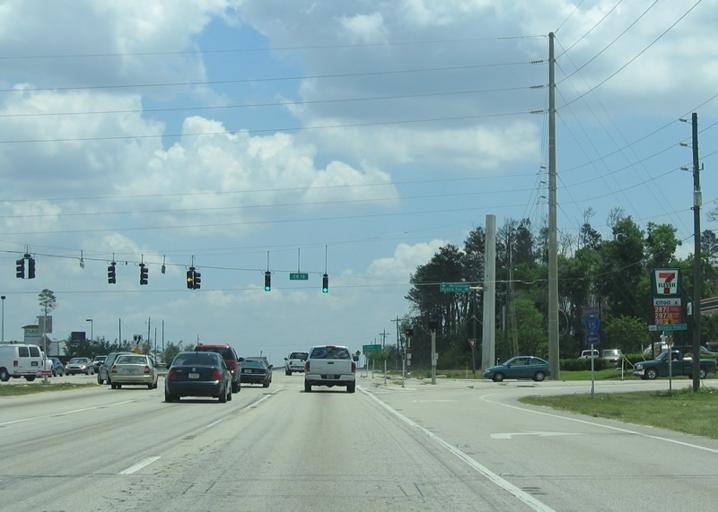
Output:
[363,344,381,354]
[439,283,471,294]
[289,272,309,281]
[585,317,599,343]
[649,324,687,331]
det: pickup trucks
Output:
[633,350,716,380]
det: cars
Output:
[482,354,551,384]
[597,348,625,369]
[110,354,158,389]
[48,355,65,377]
[164,351,233,404]
[670,344,708,353]
[643,341,675,355]
[238,355,274,387]
[579,349,599,361]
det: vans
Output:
[1,344,43,382]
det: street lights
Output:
[85,318,94,340]
[0,294,6,342]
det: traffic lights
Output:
[264,272,272,292]
[186,270,192,290]
[107,264,116,285]
[140,265,148,285]
[16,257,25,281]
[29,257,36,279]
[322,274,329,294]
[194,271,200,291]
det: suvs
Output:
[65,356,95,375]
[193,342,241,393]
[282,351,308,375]
[304,344,357,393]
[97,351,133,384]
[92,355,108,372]
[41,351,55,378]
